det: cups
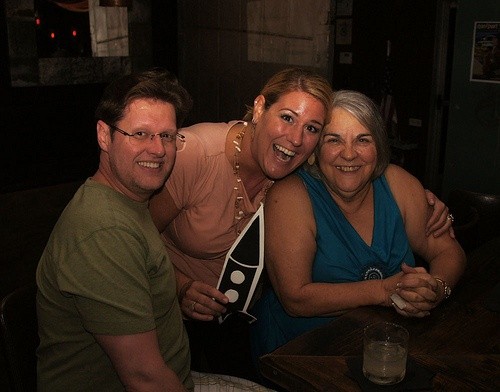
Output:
[361,321,410,386]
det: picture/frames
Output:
[469,21,500,84]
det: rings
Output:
[446,213,454,225]
[191,302,197,311]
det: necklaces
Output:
[232,120,276,238]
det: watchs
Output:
[435,274,452,307]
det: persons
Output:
[148,66,456,384]
[36,69,275,392]
[248,90,467,352]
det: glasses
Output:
[103,121,186,151]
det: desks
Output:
[255,231,500,392]
